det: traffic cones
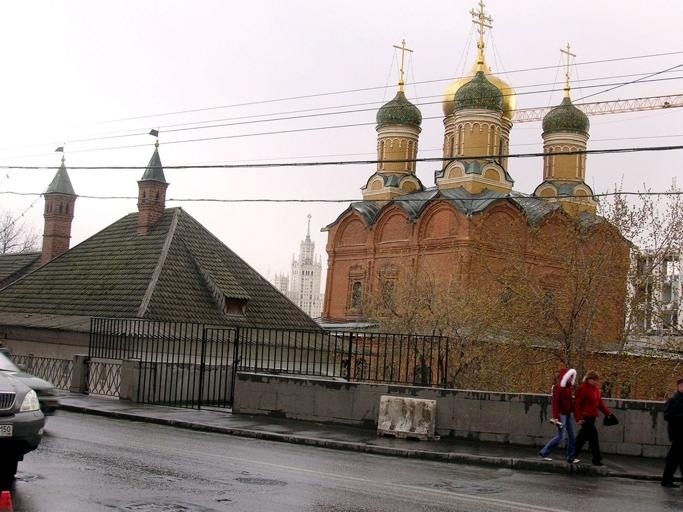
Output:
[0,490,13,512]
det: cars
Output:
[0,365,44,484]
[1,351,61,418]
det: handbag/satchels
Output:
[603,414,619,426]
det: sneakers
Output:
[662,483,680,488]
[541,456,605,467]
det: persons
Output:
[536,366,581,464]
[660,379,683,489]
[565,370,613,469]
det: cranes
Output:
[503,91,682,125]
